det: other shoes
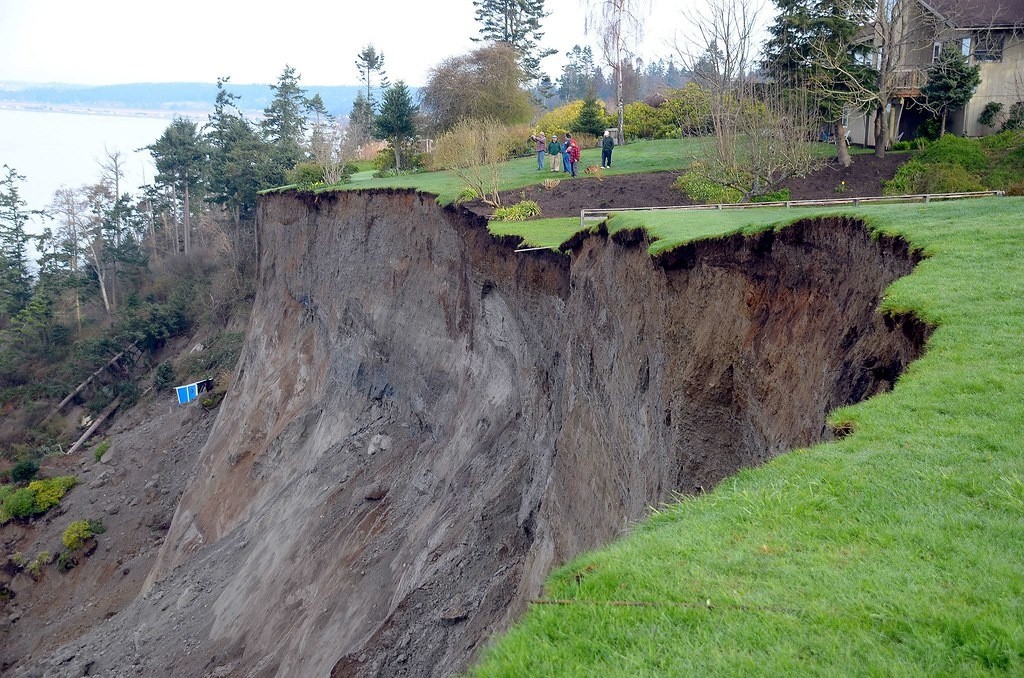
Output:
[600,167,605,168]
[607,166,610,168]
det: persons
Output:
[560,133,571,173]
[548,135,562,172]
[600,130,614,170]
[566,139,580,178]
[531,131,546,170]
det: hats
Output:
[540,132,544,135]
[552,135,557,137]
